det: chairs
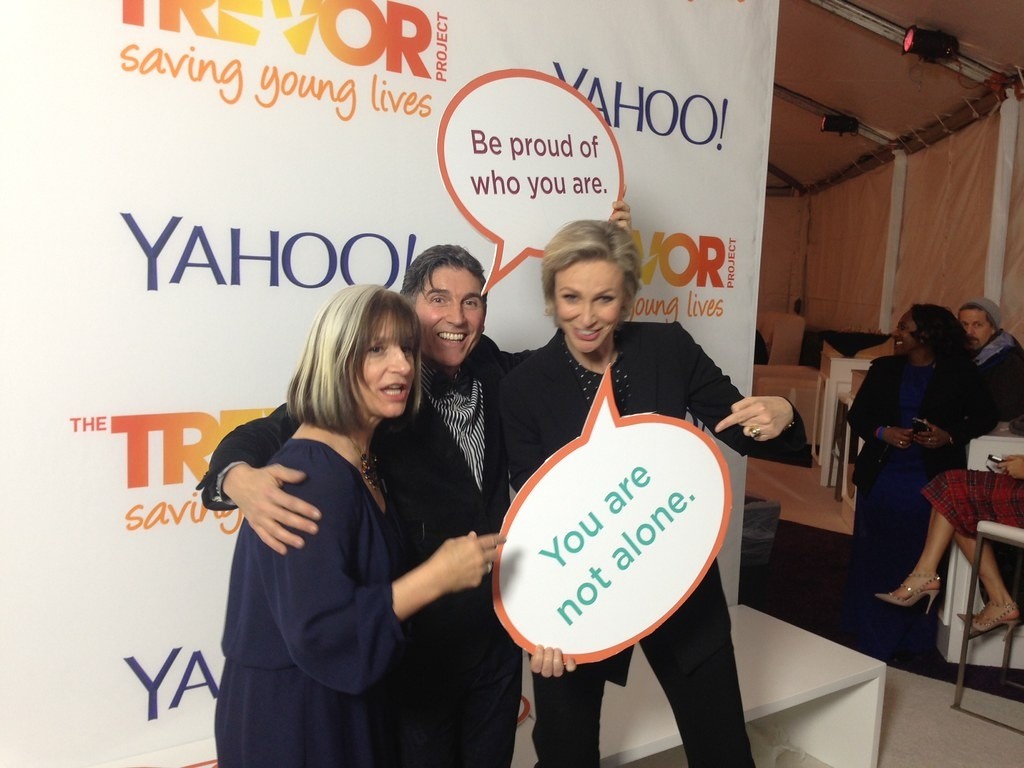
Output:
[751,312,806,364]
[951,519,1024,738]
[826,391,848,499]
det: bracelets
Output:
[783,417,799,432]
[949,436,953,445]
[874,425,891,463]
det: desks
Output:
[820,355,877,492]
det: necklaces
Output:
[344,427,383,491]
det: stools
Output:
[753,364,823,443]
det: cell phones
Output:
[911,416,927,435]
[987,454,1008,463]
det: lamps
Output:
[899,25,960,63]
[819,114,858,137]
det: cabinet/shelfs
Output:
[934,432,1023,668]
[514,604,888,768]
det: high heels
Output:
[957,598,1022,642]
[875,573,941,615]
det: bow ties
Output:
[430,371,472,400]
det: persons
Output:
[202,183,807,768]
[845,297,1024,641]
[754,330,768,365]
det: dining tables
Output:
[809,332,899,464]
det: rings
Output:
[487,560,493,575]
[749,424,761,437]
[929,436,932,442]
[899,440,903,445]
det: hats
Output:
[971,297,1002,332]
